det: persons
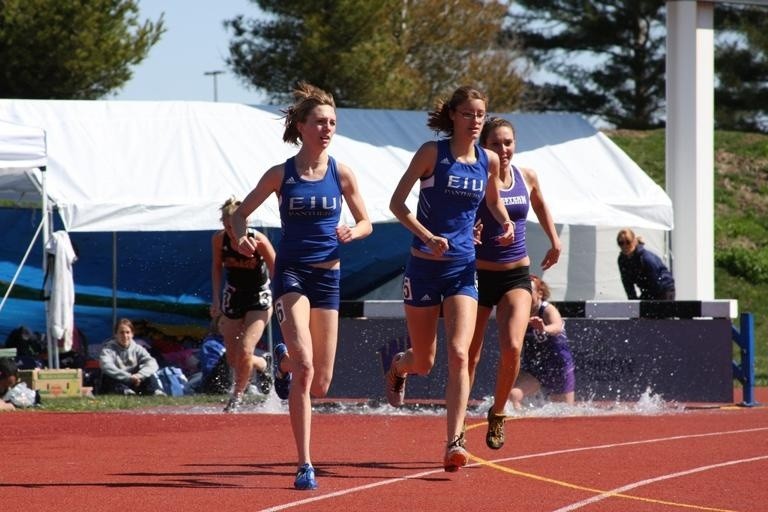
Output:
[0,356,19,414]
[231,78,375,491]
[99,318,167,398]
[467,115,562,449]
[388,84,516,477]
[200,311,268,394]
[616,228,675,303]
[508,274,577,410]
[210,194,277,413]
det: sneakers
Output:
[155,389,167,398]
[274,343,292,400]
[486,406,507,449]
[444,436,468,472]
[459,422,466,447]
[294,462,316,490]
[223,396,243,412]
[257,353,273,394]
[387,352,407,408]
[123,388,136,397]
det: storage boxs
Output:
[18,368,82,398]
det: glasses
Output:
[454,108,489,122]
[618,240,633,246]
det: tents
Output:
[1,98,673,369]
